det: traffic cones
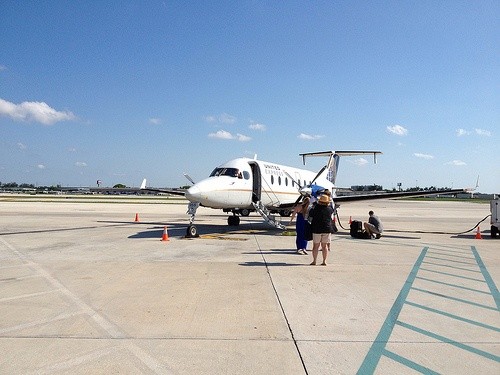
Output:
[348,216,352,224]
[134,213,139,221]
[476,226,482,239]
[159,225,170,241]
[333,215,337,223]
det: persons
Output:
[364,210,383,240]
[290,190,336,266]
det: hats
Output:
[317,195,332,203]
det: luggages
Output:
[349,220,362,237]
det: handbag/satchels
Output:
[302,221,313,242]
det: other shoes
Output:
[297,249,308,255]
[375,234,382,239]
[310,261,316,265]
[320,262,326,265]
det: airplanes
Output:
[139,149,481,238]
